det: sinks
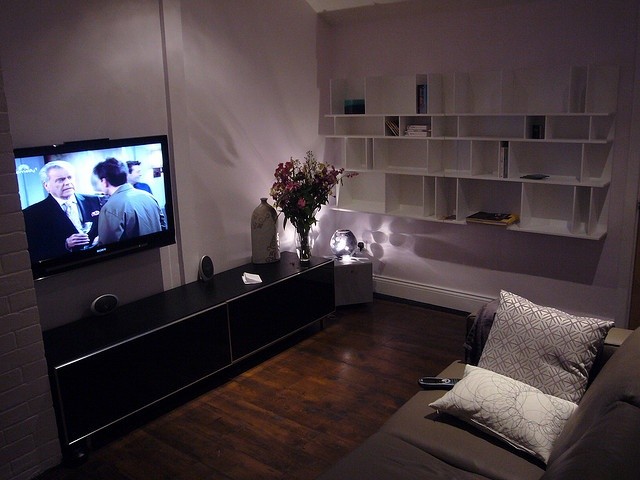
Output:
[331,294,639,480]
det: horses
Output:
[418,375,463,389]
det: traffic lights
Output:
[428,363,579,465]
[478,289,614,405]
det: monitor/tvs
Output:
[499,147,504,177]
[532,125,542,139]
[466,211,519,226]
[417,84,427,114]
[385,119,399,136]
[503,147,508,178]
[404,124,431,137]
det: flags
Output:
[295,227,314,262]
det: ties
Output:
[12,134,176,280]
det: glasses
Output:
[329,229,358,257]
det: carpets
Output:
[65,201,82,229]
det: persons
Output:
[23,161,102,263]
[92,157,162,246]
[126,161,154,197]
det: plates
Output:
[43,251,338,463]
[323,64,618,243]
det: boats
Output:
[90,293,119,315]
[199,254,215,282]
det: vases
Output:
[270,150,359,226]
